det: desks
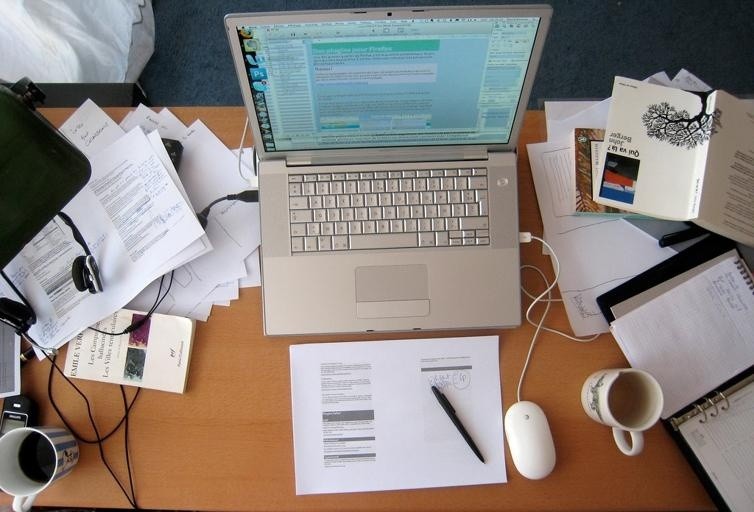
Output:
[1,107,717,512]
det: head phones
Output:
[0,212,104,333]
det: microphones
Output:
[21,332,59,356]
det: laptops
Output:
[224,4,554,335]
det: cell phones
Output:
[0,396,33,438]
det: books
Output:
[525,62,754,511]
[62,307,198,396]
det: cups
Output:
[580,368,663,458]
[0,424,79,512]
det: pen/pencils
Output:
[431,385,484,463]
[659,227,708,247]
[20,346,36,367]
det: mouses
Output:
[505,402,556,482]
